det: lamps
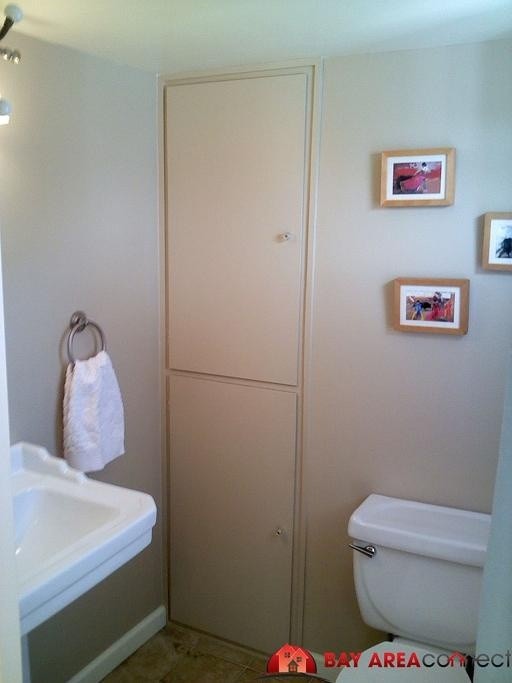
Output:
[0,1,27,126]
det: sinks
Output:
[1,442,158,636]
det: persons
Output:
[409,295,453,322]
[498,238,512,258]
[414,162,431,193]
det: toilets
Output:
[333,491,490,682]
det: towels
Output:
[59,351,130,476]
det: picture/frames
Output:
[483,211,512,273]
[392,277,469,336]
[377,145,456,209]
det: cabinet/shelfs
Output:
[161,371,299,665]
[164,61,312,386]
